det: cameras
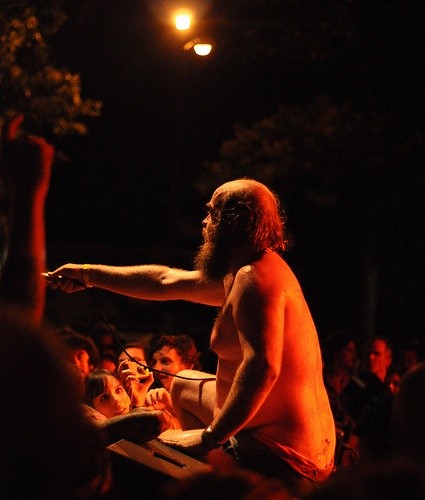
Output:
[125,360,150,379]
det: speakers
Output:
[104,435,214,500]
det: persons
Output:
[172,326,201,358]
[0,318,170,500]
[325,328,425,500]
[0,68,54,333]
[46,179,336,496]
[131,325,164,363]
[84,369,177,419]
[93,322,118,361]
[118,342,150,376]
[45,326,100,379]
[118,334,204,409]
[91,353,118,379]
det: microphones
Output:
[39,268,86,287]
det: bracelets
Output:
[82,264,93,288]
